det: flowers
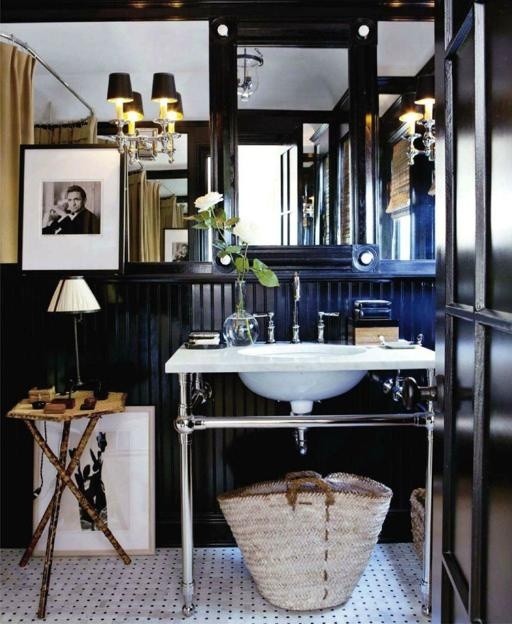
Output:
[183,191,279,343]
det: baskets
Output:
[410,488,426,567]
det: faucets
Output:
[287,268,304,345]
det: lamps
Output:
[47,277,102,389]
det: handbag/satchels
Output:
[217,470,394,611]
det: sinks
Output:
[236,341,370,408]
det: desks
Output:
[6,389,132,620]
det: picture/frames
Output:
[17,142,127,279]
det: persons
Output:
[43,185,99,234]
[172,243,188,260]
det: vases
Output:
[222,276,260,347]
